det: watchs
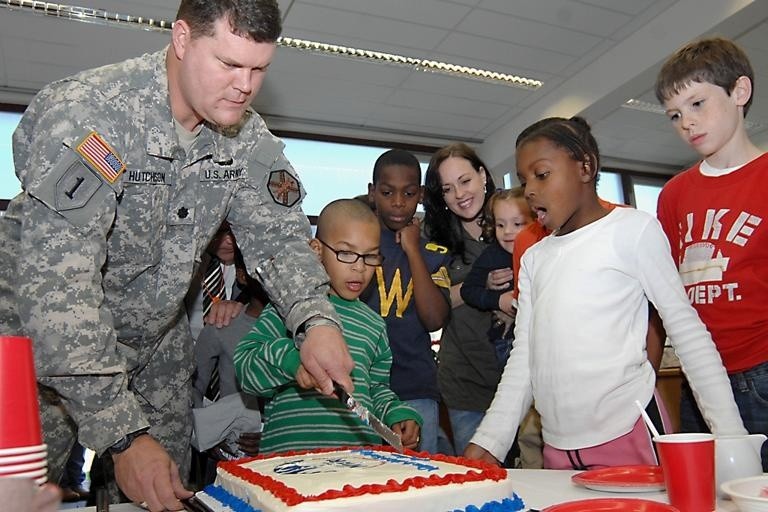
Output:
[110,430,149,451]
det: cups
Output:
[0,336,48,489]
[652,432,716,512]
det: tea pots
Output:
[714,434,768,500]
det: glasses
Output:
[311,235,385,268]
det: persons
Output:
[464,116,750,469]
[58,441,91,503]
[0,0,357,512]
[513,175,666,469]
[356,148,451,453]
[182,200,424,460]
[460,188,538,369]
[420,143,520,469]
[646,37,768,474]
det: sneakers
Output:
[58,482,92,504]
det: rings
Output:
[140,502,148,508]
[417,437,419,442]
[497,321,501,325]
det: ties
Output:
[201,257,230,323]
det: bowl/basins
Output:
[720,475,768,512]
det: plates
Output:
[571,464,666,493]
[541,498,679,512]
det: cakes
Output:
[195,445,525,512]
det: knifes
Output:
[333,381,405,453]
